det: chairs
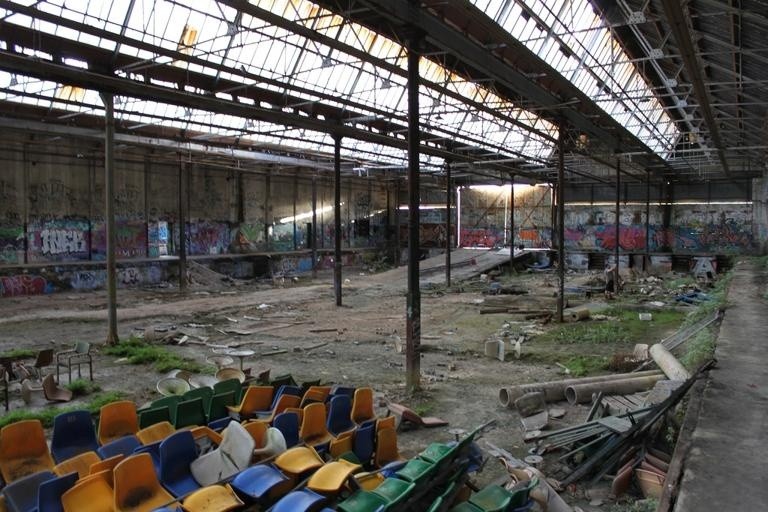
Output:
[611,438,671,498]
